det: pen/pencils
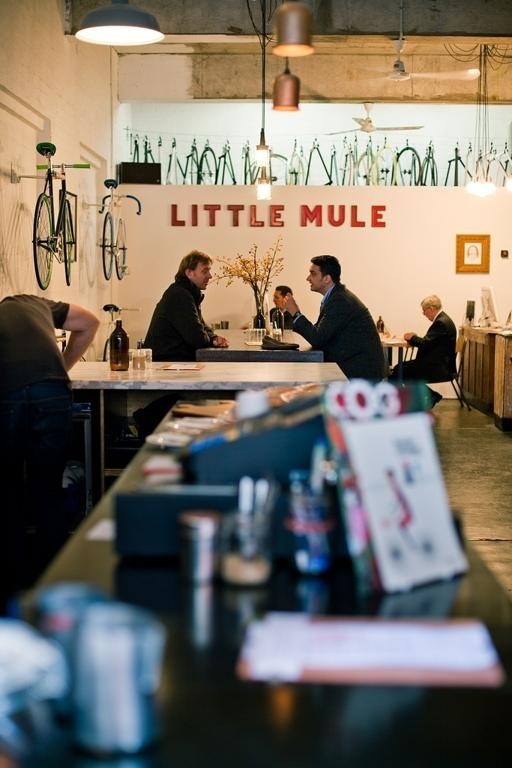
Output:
[289,440,342,577]
[227,473,276,563]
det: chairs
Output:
[421,333,471,412]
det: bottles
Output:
[253,308,265,329]
[272,307,284,336]
[109,319,130,371]
[377,316,384,333]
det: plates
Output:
[143,431,191,449]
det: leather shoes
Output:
[428,392,443,408]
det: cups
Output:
[0,490,334,768]
[245,329,282,343]
[132,349,153,370]
[221,321,229,329]
[248,319,253,329]
[237,391,270,422]
[466,300,475,321]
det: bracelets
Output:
[293,311,301,320]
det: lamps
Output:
[255,0,273,200]
[71,0,165,49]
[443,43,512,195]
[269,0,316,60]
[254,0,273,170]
[270,56,304,113]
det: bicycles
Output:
[94,175,143,282]
[30,140,90,289]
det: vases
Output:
[253,305,266,328]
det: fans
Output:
[323,101,425,139]
[343,0,481,84]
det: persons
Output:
[392,295,455,410]
[284,256,385,381]
[261,286,293,330]
[143,249,229,361]
[0,293,99,572]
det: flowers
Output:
[206,232,287,302]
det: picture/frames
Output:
[455,233,491,274]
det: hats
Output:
[261,335,300,350]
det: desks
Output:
[377,332,414,383]
[195,320,324,363]
